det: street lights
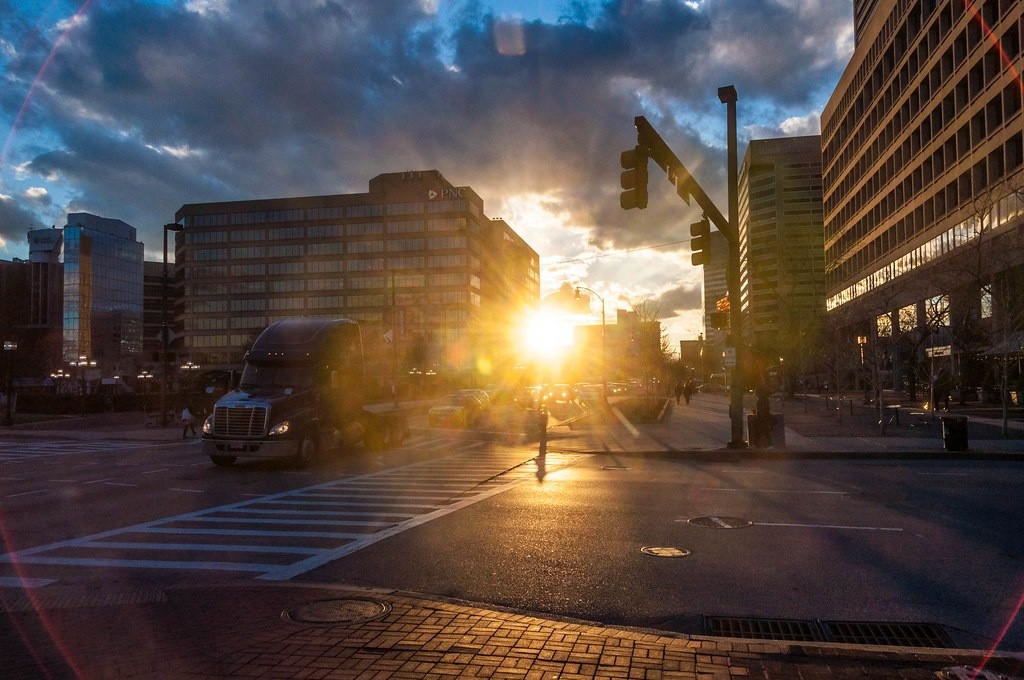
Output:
[135,369,155,412]
[161,224,184,430]
[179,360,200,405]
[70,355,98,418]
[112,375,120,395]
[3,338,19,425]
[50,368,70,398]
[575,286,609,412]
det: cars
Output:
[428,378,657,426]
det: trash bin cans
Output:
[939,415,969,450]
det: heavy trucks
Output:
[200,315,405,472]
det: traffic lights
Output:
[690,217,711,266]
[709,312,728,329]
[620,149,647,209]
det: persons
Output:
[943,395,951,413]
[934,395,940,412]
[181,401,199,438]
[683,382,692,403]
[674,383,682,403]
